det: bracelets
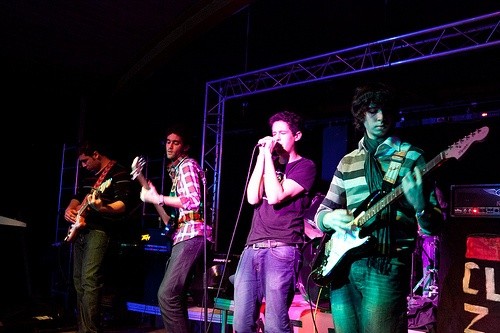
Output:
[417,208,431,218]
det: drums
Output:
[297,238,331,310]
[304,193,326,240]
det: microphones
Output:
[256,144,262,147]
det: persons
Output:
[140,128,216,333]
[232,111,316,333]
[65,142,131,333]
[314,80,445,333]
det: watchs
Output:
[157,194,164,208]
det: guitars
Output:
[131,152,177,243]
[309,125,490,290]
[63,178,113,243]
